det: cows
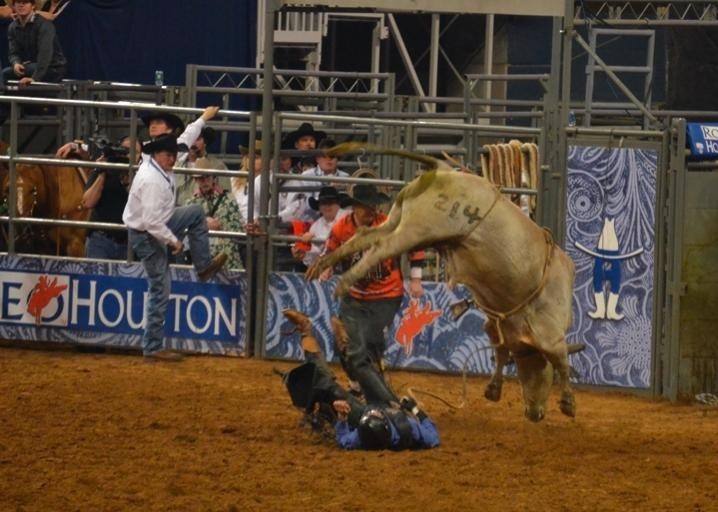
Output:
[304,142,586,422]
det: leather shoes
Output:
[143,347,184,361]
[199,251,227,283]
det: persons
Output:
[174,127,229,204]
[1,1,69,129]
[56,135,142,260]
[279,307,440,452]
[140,106,220,188]
[230,123,358,283]
[122,105,228,361]
[316,183,428,397]
[187,160,244,269]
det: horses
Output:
[0,140,96,257]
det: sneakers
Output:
[348,388,365,403]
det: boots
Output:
[330,315,352,352]
[280,309,322,354]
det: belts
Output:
[97,230,127,244]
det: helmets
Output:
[358,409,392,452]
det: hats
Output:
[269,122,342,162]
[238,139,264,153]
[3,0,46,11]
[200,126,216,145]
[190,157,226,178]
[142,132,190,154]
[339,182,390,208]
[308,186,353,211]
[137,108,186,132]
